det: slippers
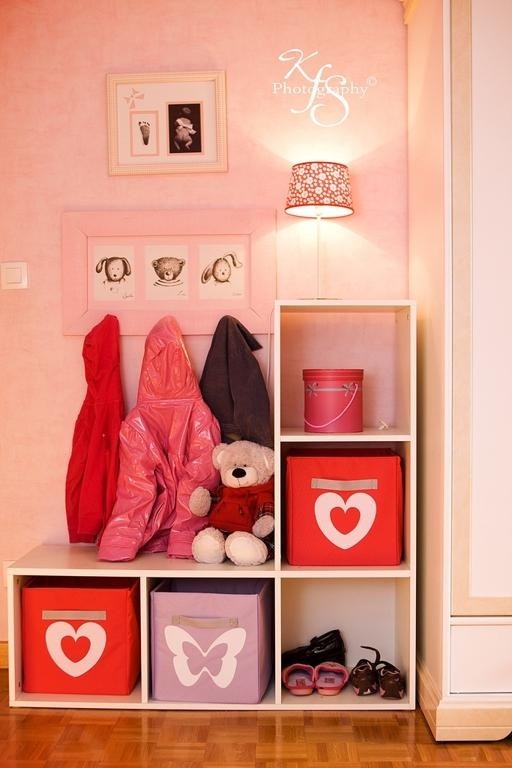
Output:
[315,662,349,696]
[282,663,315,695]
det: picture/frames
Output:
[61,207,275,337]
[106,72,228,173]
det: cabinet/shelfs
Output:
[404,0,512,743]
[7,301,418,714]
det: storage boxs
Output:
[20,579,140,694]
[285,450,403,567]
[150,579,272,705]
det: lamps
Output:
[284,163,354,299]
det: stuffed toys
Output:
[189,437,276,565]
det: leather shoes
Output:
[282,629,346,666]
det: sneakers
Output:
[350,657,379,696]
[378,666,405,700]
